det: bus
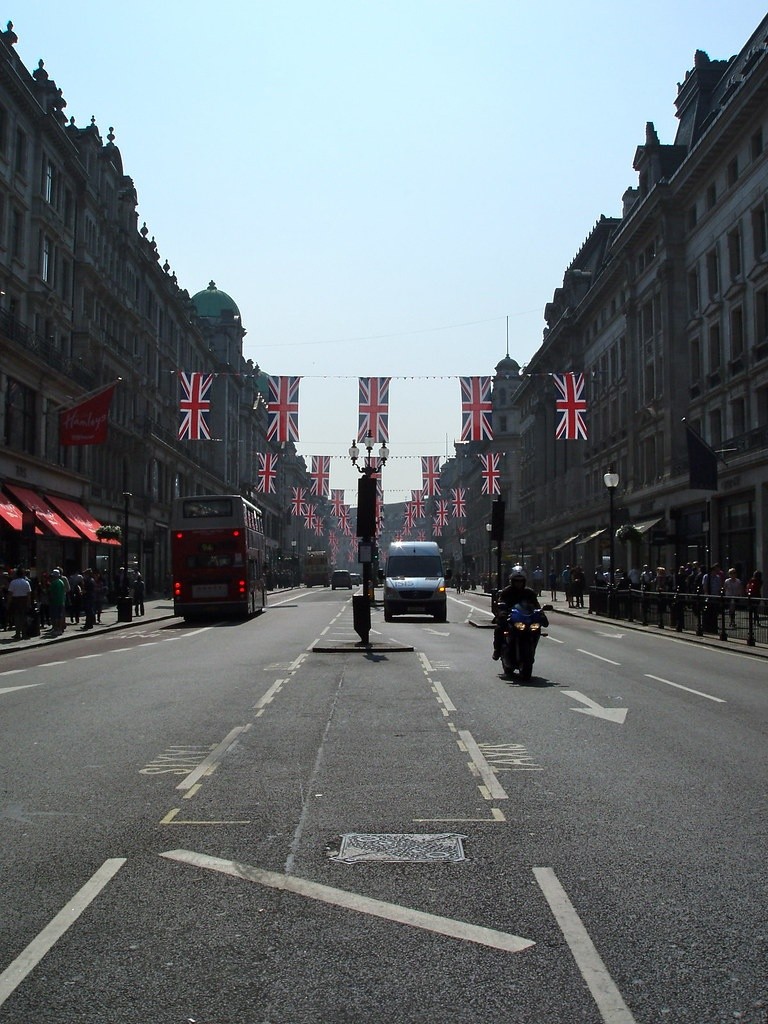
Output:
[170,493,268,622]
[305,550,333,588]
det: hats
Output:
[52,569,60,576]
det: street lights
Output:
[460,536,467,575]
[603,463,621,616]
[349,429,389,604]
[485,520,495,582]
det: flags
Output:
[266,376,299,443]
[422,457,442,496]
[431,489,466,537]
[329,529,358,564]
[59,386,114,446]
[310,456,329,496]
[176,372,212,440]
[256,454,279,495]
[480,453,502,496]
[685,428,719,491]
[356,378,389,444]
[291,486,326,537]
[553,375,588,440]
[460,377,493,441]
[331,490,352,537]
[365,458,386,563]
[395,490,426,541]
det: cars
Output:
[331,569,353,590]
[350,572,364,586]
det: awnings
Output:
[5,484,81,538]
[575,527,608,544]
[1,491,44,535]
[634,519,662,534]
[45,494,122,546]
[552,535,580,552]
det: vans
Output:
[378,540,453,622]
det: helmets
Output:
[510,571,527,587]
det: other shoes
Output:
[492,650,500,661]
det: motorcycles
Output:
[495,598,553,681]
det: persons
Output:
[509,563,587,608]
[453,570,487,595]
[0,561,145,640]
[492,575,549,661]
[596,559,764,629]
